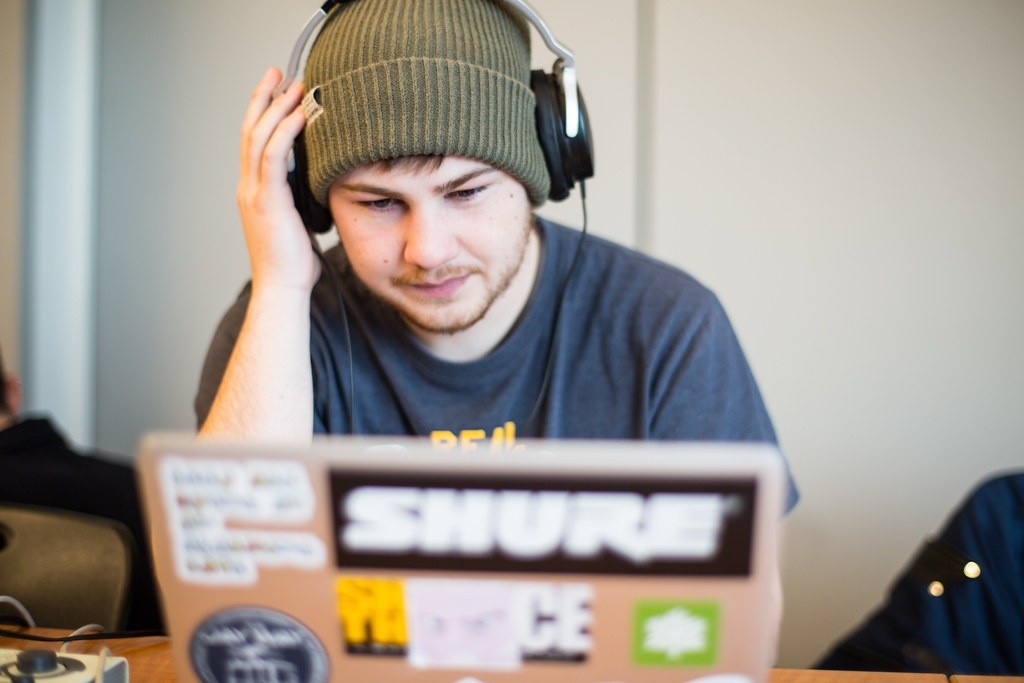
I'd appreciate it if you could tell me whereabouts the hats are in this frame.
[293,0,550,207]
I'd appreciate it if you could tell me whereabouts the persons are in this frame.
[193,0,799,520]
[811,466,1024,676]
[0,355,167,637]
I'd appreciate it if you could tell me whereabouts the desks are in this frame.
[0,625,1024,683]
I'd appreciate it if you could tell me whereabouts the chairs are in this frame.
[0,503,132,633]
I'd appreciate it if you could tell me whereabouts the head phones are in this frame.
[282,0,596,235]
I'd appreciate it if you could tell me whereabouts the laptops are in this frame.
[134,429,784,683]
